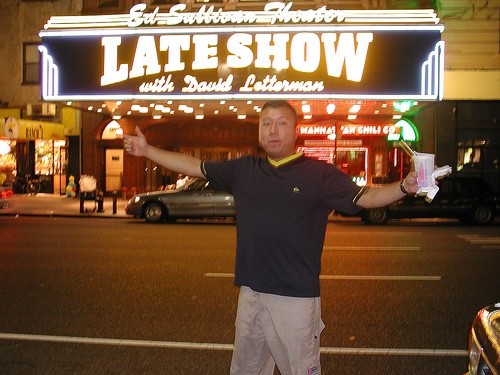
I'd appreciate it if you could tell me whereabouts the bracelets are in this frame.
[400,178,416,197]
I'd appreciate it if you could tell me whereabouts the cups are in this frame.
[412,154,435,192]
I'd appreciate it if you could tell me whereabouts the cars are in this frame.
[0,186,14,209]
[125,167,236,224]
[467,302,500,374]
[338,170,500,224]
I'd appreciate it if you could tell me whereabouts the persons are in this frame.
[116,101,449,375]
